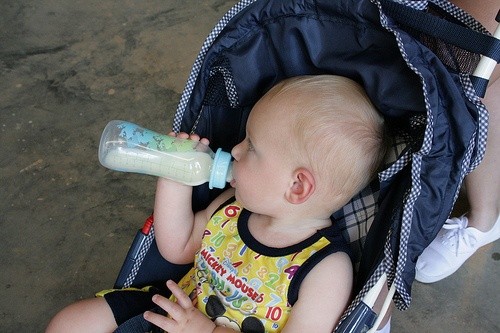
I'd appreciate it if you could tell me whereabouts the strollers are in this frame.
[113,0,500,333]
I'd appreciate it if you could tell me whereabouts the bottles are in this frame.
[98,119,233,189]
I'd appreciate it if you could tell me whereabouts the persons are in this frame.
[414,0,500,284]
[45,74,386,333]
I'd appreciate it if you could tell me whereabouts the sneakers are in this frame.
[415,209,500,283]
